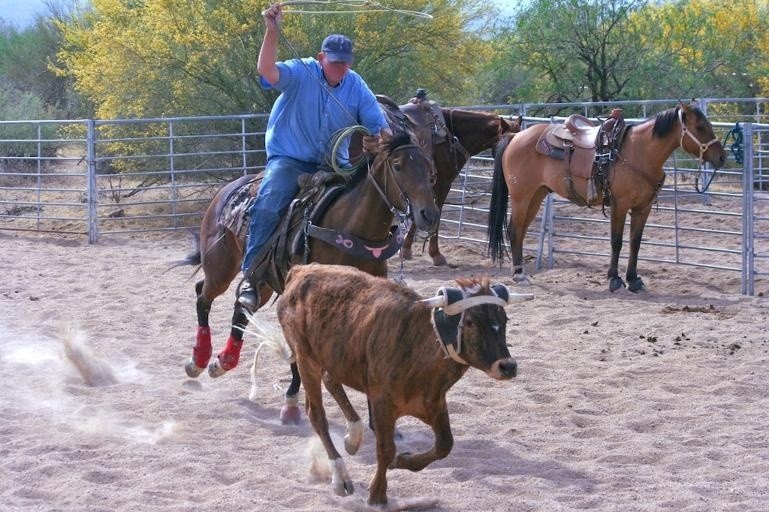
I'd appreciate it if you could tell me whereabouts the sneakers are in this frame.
[238,277,258,310]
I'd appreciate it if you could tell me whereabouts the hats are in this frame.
[322,33,354,64]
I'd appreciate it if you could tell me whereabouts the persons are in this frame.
[235,4,389,308]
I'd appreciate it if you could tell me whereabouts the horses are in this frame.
[160,128,442,443]
[485,95,727,298]
[401,107,523,266]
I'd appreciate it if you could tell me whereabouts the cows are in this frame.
[233,261,535,510]
